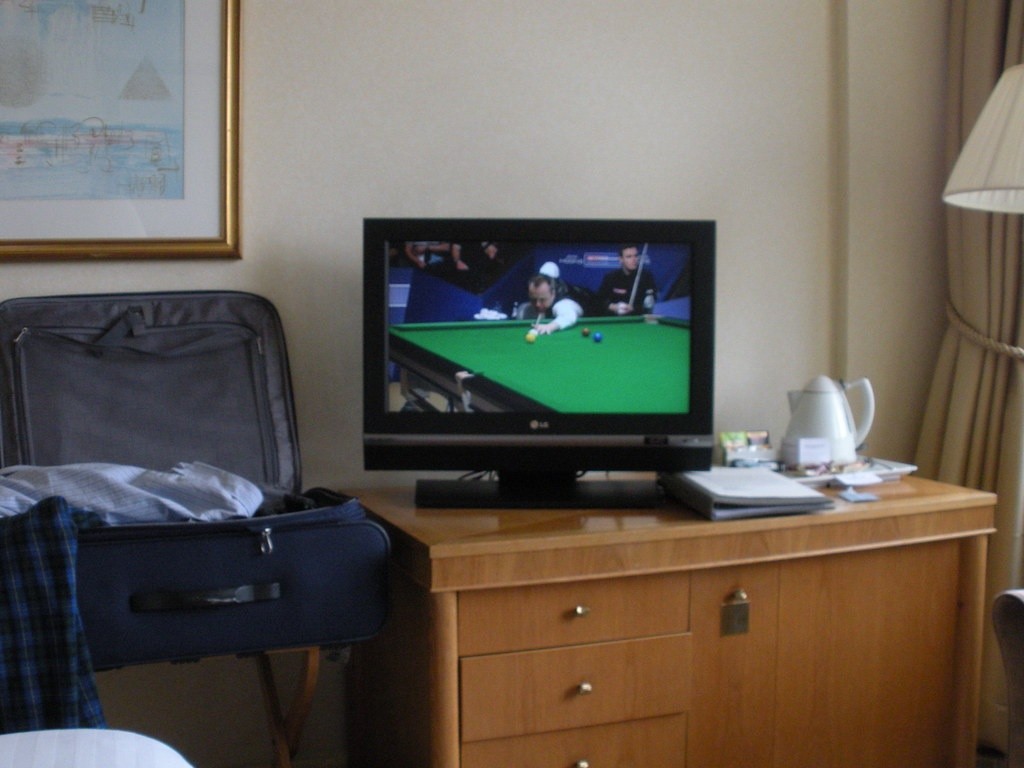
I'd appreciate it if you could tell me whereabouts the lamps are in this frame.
[941,64,1024,213]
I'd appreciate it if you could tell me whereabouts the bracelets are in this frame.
[455,260,460,263]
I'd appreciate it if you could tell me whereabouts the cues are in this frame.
[535,312,542,324]
[629,243,648,303]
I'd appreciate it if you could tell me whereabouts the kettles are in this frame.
[781,375,876,465]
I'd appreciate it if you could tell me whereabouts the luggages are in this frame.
[0,291,394,671]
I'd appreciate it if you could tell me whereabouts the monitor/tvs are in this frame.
[362,217,716,510]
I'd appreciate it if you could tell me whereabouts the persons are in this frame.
[595,242,658,317]
[528,262,605,335]
[404,241,537,294]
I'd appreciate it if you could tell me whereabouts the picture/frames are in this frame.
[0,0,241,261]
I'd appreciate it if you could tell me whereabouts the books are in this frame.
[657,463,837,521]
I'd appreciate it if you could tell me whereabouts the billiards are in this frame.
[526,329,538,342]
[582,328,590,336]
[593,332,603,342]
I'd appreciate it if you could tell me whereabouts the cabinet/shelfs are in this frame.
[348,470,998,768]
